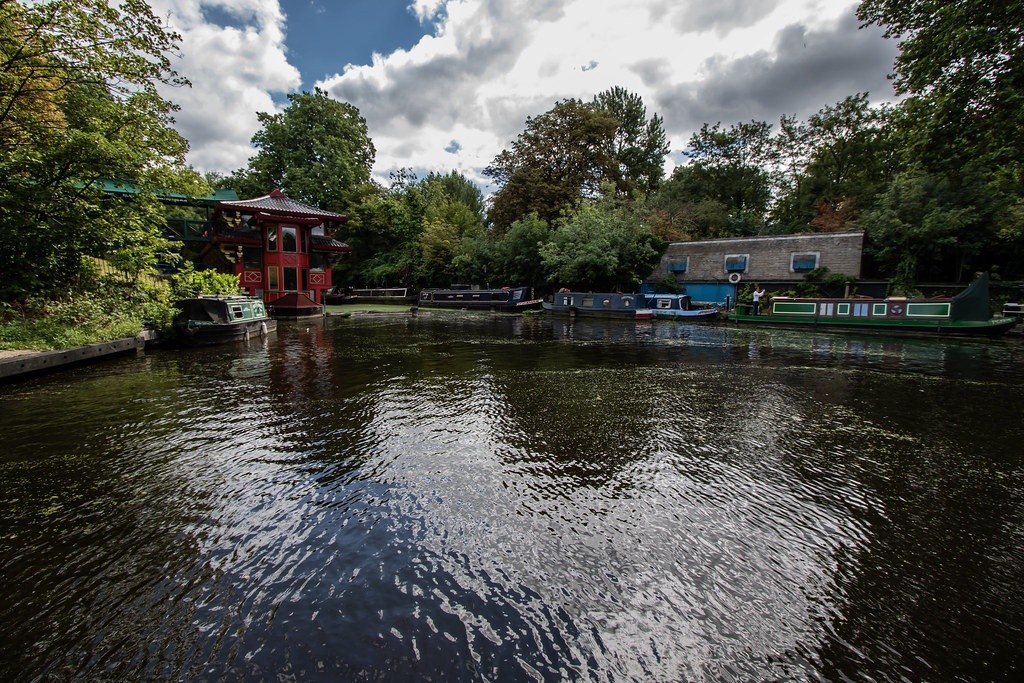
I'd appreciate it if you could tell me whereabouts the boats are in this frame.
[727,270,1019,338]
[417,286,544,311]
[541,290,720,323]
[174,291,278,346]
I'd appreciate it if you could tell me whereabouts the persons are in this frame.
[753,286,766,315]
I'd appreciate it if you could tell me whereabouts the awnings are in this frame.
[794,255,816,268]
[725,256,746,270]
[670,258,687,270]
